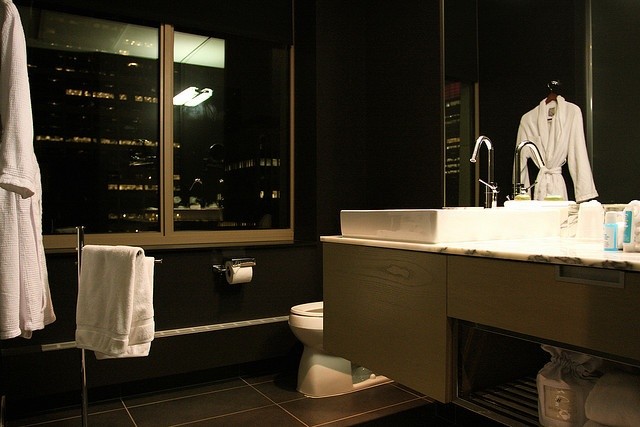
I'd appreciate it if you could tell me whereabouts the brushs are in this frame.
[233,258,256,270]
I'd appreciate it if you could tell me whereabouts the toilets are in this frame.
[288,301,395,399]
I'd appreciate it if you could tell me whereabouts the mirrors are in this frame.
[442,0,640,207]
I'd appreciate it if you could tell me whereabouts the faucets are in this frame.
[514,140,546,199]
[469,136,500,207]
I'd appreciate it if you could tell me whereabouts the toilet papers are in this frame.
[225,261,253,285]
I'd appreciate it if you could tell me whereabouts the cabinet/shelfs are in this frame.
[446,248,639,427]
[321,235,451,404]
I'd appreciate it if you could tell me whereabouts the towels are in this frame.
[94,256,155,360]
[76,244,154,358]
[582,369,639,427]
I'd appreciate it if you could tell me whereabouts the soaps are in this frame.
[513,195,532,201]
[546,195,563,201]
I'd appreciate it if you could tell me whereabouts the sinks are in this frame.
[339,208,561,244]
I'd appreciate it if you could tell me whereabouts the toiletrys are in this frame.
[602,210,619,251]
[575,198,640,252]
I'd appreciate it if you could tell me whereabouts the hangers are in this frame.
[538,79,563,109]
